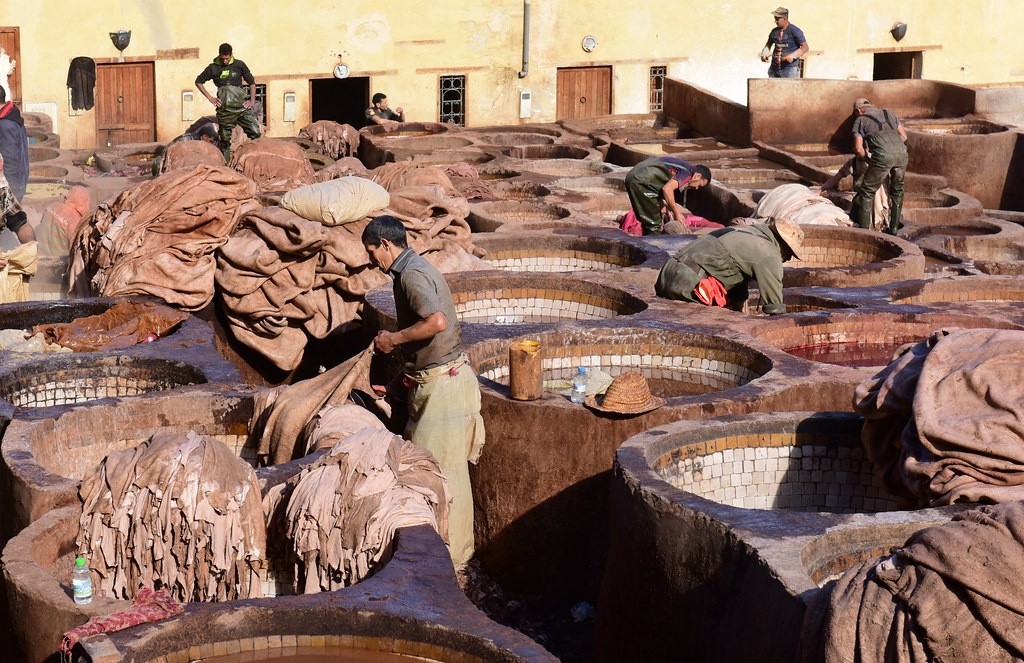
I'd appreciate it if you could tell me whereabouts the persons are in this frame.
[0,86,30,204]
[194,43,261,166]
[655,217,804,315]
[366,93,405,125]
[761,7,809,78]
[152,127,220,177]
[361,215,482,572]
[824,98,909,234]
[624,156,712,235]
[0,154,38,270]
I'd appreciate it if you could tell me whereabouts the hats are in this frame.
[771,6,789,17]
[853,97,871,114]
[771,217,805,262]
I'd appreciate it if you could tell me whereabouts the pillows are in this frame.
[279,175,390,226]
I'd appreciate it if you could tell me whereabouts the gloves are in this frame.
[760,47,769,63]
[784,49,803,62]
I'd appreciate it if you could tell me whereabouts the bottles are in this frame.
[72,558,93,604]
[571,367,588,403]
